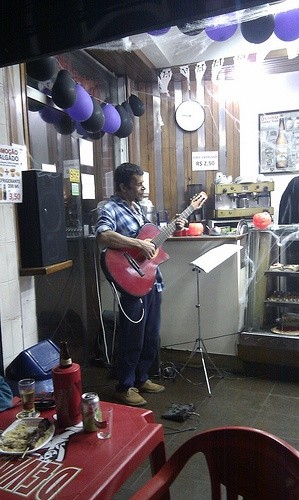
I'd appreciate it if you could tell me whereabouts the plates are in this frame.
[0,417,55,456]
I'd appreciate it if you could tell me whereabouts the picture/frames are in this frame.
[258,109,299,175]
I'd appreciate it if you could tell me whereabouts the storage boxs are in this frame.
[6,338,61,381]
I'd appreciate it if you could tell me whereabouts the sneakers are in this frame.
[115,387,147,406]
[139,380,165,393]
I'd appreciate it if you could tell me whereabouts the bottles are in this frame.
[52,341,84,427]
[276,118,288,168]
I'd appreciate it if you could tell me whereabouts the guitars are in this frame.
[99,190,208,298]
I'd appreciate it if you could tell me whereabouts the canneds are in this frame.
[81,392,102,432]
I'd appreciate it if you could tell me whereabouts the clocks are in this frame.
[174,100,206,133]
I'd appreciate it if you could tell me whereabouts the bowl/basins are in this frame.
[209,228,222,236]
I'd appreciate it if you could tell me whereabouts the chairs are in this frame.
[127,425,299,500]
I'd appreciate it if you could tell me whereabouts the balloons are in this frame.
[240,3,274,44]
[274,8,299,42]
[25,55,146,141]
[177,19,204,36]
[147,27,170,36]
[203,12,238,42]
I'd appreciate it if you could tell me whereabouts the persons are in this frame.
[94,163,189,406]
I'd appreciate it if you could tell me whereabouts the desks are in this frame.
[0,399,171,500]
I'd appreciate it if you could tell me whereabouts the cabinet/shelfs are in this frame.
[237,224,299,378]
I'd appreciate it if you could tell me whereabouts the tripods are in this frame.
[178,244,238,397]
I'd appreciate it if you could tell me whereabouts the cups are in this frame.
[92,404,113,441]
[17,379,36,417]
[189,222,204,236]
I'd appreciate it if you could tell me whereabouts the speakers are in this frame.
[15,170,69,269]
[6,337,61,383]
